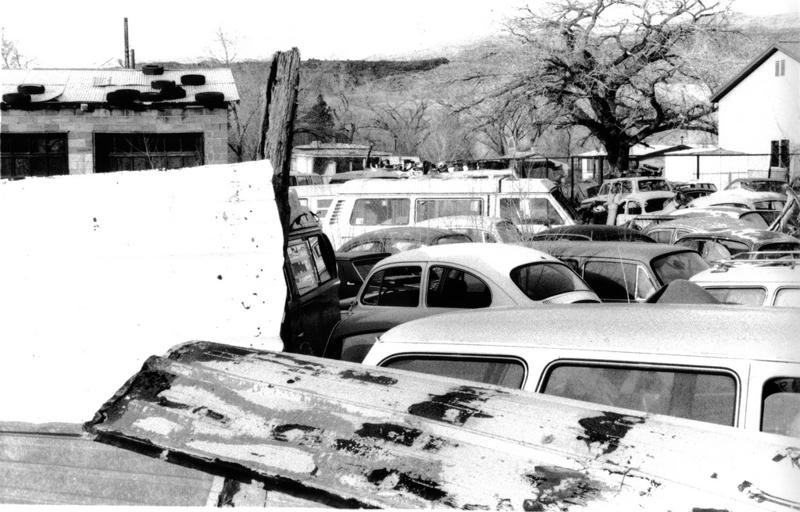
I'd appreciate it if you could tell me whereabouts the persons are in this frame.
[367,154,489,175]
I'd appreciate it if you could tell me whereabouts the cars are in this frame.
[348,241,599,304]
[278,151,800,363]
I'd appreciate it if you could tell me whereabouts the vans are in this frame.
[318,174,585,256]
[359,301,799,442]
[523,239,710,302]
